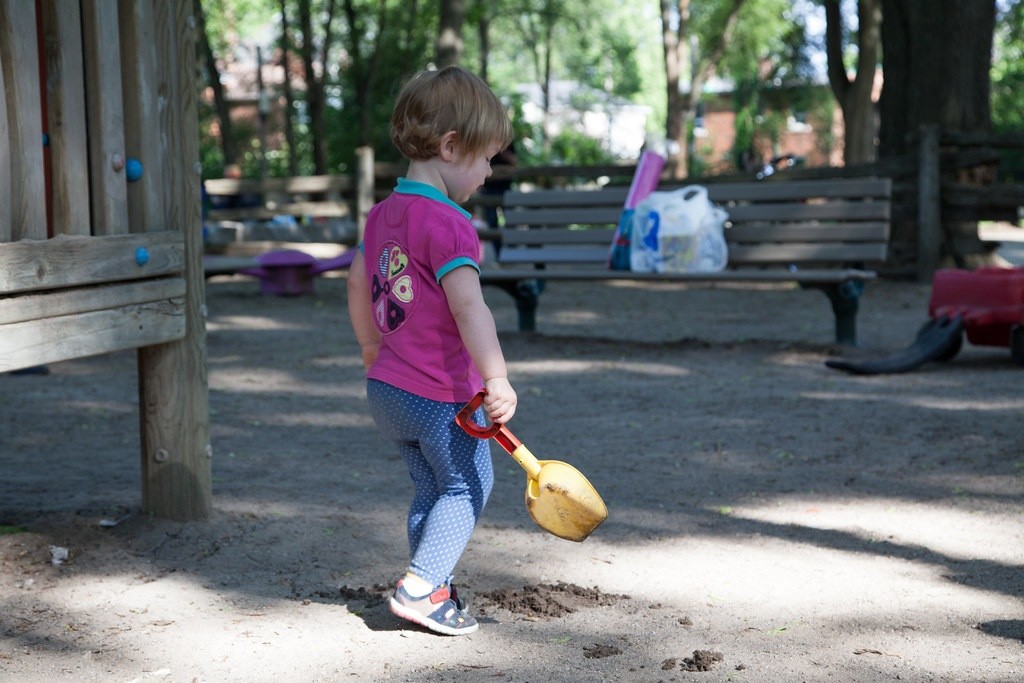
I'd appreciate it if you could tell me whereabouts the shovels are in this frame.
[454,387,609,543]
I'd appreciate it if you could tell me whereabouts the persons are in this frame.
[347,67,518,635]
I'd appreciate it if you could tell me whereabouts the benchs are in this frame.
[479,176,895,347]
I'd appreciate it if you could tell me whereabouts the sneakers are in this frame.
[386,577,479,635]
[396,566,469,614]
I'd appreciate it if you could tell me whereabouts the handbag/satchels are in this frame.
[628,183,729,277]
